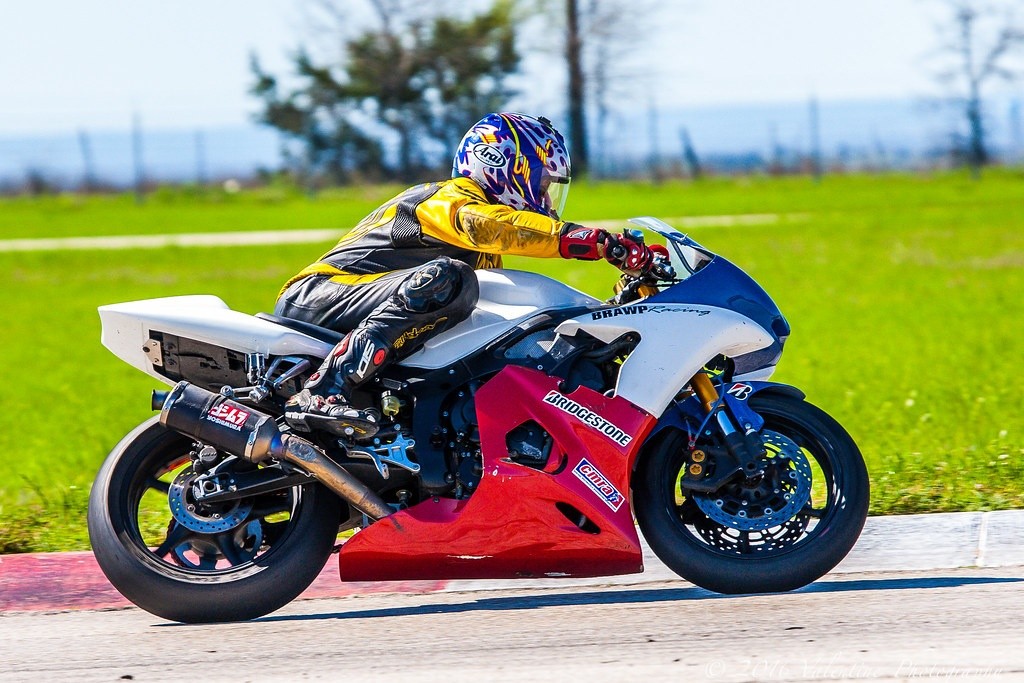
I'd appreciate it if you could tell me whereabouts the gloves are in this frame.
[558,220,655,276]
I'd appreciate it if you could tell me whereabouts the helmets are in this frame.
[451,111,572,221]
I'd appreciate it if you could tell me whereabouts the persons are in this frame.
[274,111,654,440]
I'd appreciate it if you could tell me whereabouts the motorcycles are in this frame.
[83,215,872,625]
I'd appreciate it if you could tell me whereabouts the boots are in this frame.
[284,328,399,440]
